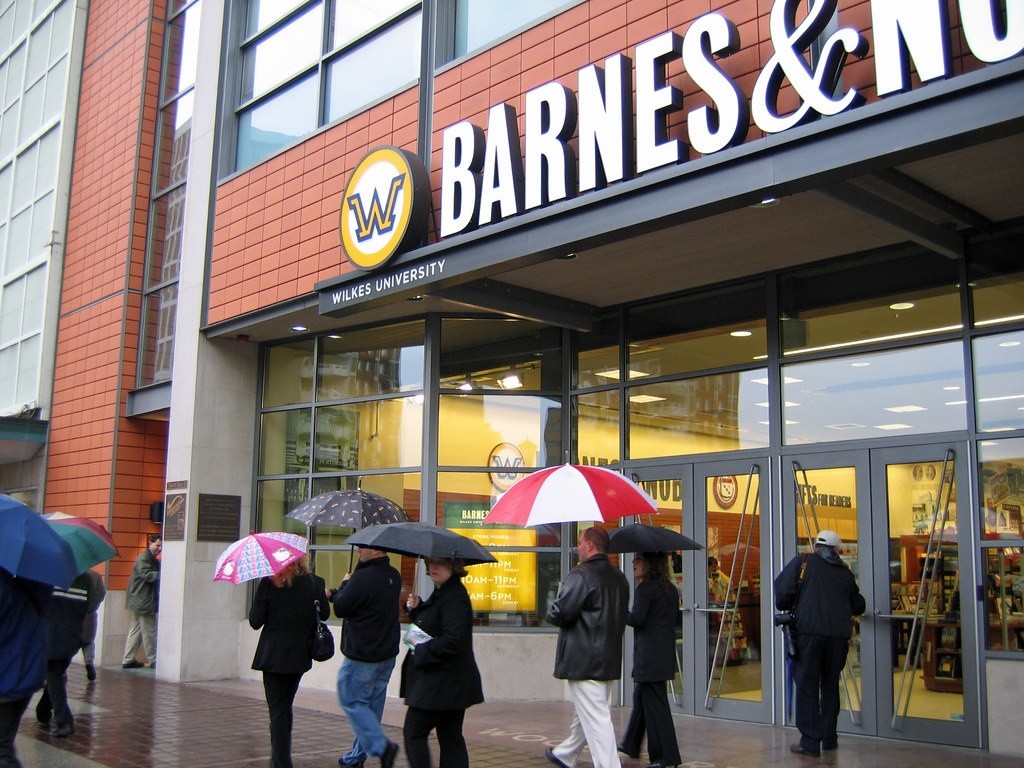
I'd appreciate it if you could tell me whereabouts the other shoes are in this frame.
[38,721,50,728]
[617,743,638,760]
[647,762,678,768]
[51,723,74,736]
[381,741,399,768]
[546,748,569,768]
[123,660,144,668]
[338,758,363,768]
[86,663,96,681]
[151,662,156,668]
[821,743,837,750]
[791,744,817,757]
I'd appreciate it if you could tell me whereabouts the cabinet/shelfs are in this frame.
[918,618,1024,693]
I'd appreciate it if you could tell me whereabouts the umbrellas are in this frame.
[574,523,704,554]
[481,462,660,571]
[281,476,413,574]
[213,529,310,586]
[343,521,499,593]
[0,493,80,591]
[42,511,121,575]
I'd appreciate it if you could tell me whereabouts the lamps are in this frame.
[497,365,525,389]
[149,500,162,524]
[454,372,479,398]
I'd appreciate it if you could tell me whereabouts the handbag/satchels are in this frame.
[312,622,334,662]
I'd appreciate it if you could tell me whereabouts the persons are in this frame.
[36,572,95,736]
[122,535,163,667]
[399,555,485,768]
[772,530,865,757]
[545,527,630,768]
[248,553,331,768]
[81,569,107,681]
[0,565,49,768]
[617,554,682,768]
[324,547,402,768]
[707,556,733,594]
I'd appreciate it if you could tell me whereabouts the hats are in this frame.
[816,530,841,546]
